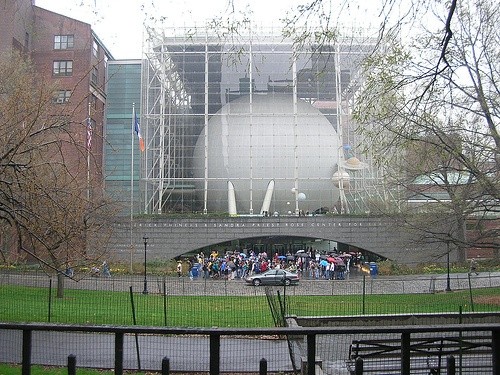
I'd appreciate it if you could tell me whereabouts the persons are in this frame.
[68,263,73,277]
[469,258,479,276]
[177,262,183,278]
[187,247,364,280]
[101,261,111,277]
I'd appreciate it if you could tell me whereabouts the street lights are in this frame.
[142,233,149,294]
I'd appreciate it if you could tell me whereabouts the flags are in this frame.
[87,117,92,148]
[135,113,145,151]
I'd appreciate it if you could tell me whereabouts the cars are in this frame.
[245,268,300,286]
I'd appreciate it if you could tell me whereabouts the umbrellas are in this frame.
[349,252,356,254]
[327,257,336,262]
[287,256,295,260]
[320,255,328,260]
[338,255,346,257]
[240,253,247,257]
[320,261,328,266]
[278,256,286,260]
[327,250,336,252]
[344,254,352,256]
[330,253,338,256]
[301,253,308,257]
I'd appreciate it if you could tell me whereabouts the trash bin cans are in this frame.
[192,265,198,276]
[370,264,376,275]
